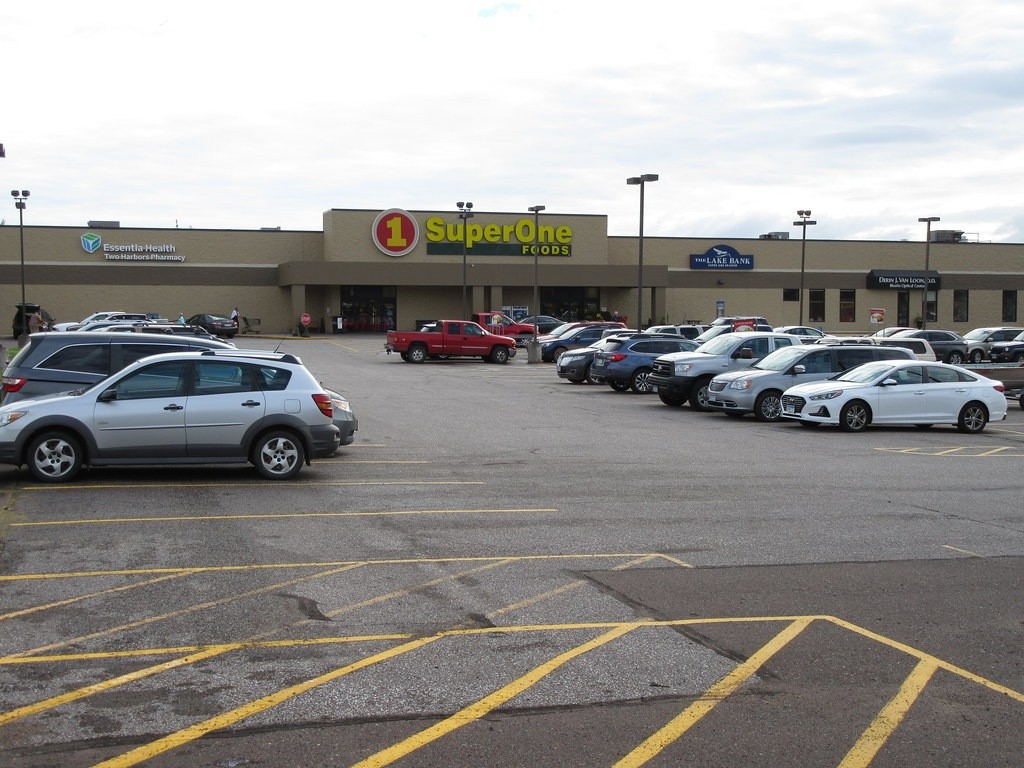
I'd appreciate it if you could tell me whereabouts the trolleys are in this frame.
[241,317,260,335]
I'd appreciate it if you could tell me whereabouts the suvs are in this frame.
[530,315,1024,423]
[0,311,358,447]
[0,348,341,484]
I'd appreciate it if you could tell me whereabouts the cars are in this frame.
[780,360,1007,434]
[185,313,238,338]
[515,316,567,334]
[12,303,56,339]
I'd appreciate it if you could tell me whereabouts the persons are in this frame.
[178,313,186,324]
[231,306,241,327]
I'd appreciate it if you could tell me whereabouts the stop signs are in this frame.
[301,313,310,325]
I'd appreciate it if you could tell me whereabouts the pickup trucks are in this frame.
[384,312,539,365]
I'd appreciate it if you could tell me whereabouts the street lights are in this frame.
[626,174,658,332]
[528,205,546,364]
[792,210,816,327]
[456,201,474,320]
[11,190,30,348]
[918,217,940,330]
